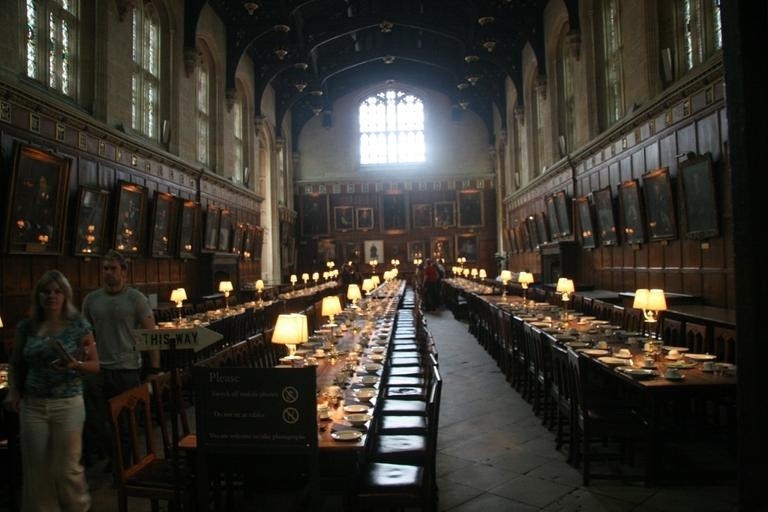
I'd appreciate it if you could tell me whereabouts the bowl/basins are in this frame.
[331,317,392,427]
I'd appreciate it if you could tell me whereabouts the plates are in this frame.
[507,301,733,379]
[332,430,362,442]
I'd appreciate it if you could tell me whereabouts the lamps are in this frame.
[311,257,534,299]
[244,0,497,117]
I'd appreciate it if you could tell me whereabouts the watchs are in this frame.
[78,360,82,367]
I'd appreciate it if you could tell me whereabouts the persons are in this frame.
[5,270,100,512]
[80,250,162,488]
[416,257,445,312]
[341,263,359,309]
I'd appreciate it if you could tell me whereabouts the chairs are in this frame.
[94,274,442,511]
[443,274,736,485]
